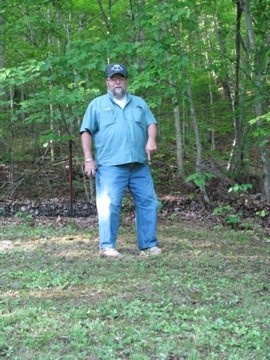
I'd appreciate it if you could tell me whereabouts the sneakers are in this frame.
[99,247,121,258]
[138,247,163,258]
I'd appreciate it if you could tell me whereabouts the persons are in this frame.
[79,64,162,259]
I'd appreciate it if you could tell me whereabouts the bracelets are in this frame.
[85,158,93,161]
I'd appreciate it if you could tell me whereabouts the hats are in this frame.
[106,64,128,79]
